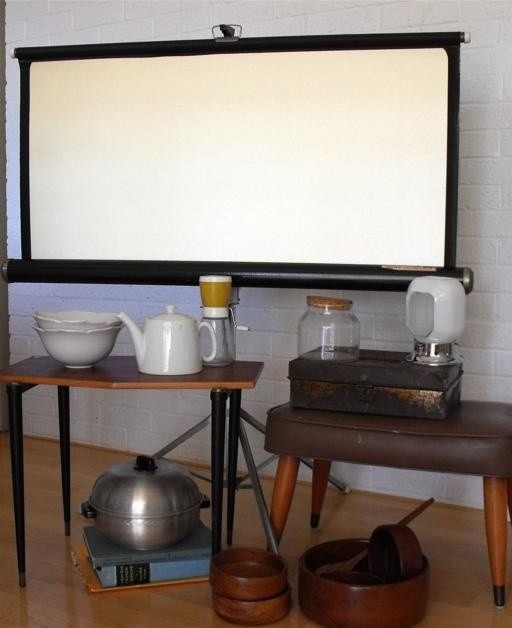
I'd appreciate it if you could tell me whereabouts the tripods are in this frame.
[149,286,350,557]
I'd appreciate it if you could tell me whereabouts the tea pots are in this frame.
[119,304,218,376]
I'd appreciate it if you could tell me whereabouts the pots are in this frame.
[80,454,212,550]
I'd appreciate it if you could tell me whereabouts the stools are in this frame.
[266,399,511,608]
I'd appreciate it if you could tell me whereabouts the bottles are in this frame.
[405,276,467,366]
[297,295,361,362]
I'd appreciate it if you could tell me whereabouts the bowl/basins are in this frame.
[31,307,128,371]
[209,523,432,628]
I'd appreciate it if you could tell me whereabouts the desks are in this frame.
[0,357,263,586]
